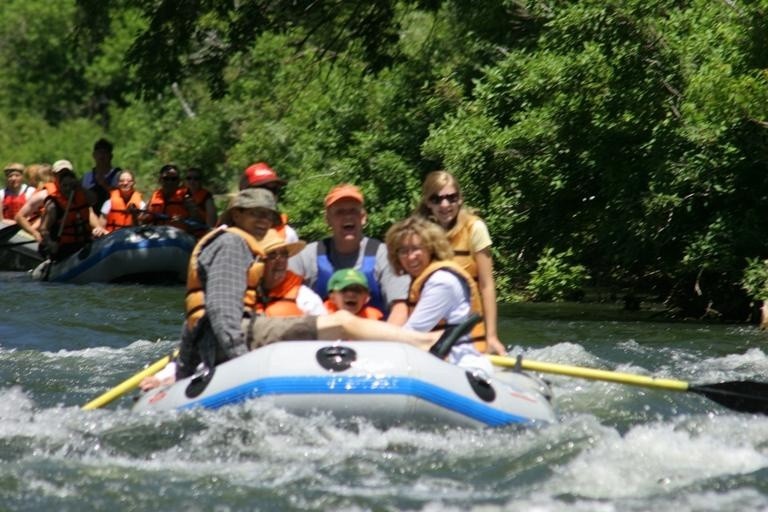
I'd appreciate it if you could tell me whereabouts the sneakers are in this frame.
[429,311,482,359]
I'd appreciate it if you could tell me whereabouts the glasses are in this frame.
[428,193,459,205]
[267,250,289,259]
[266,184,281,195]
[161,176,177,182]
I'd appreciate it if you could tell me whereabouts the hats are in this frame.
[4,163,24,174]
[239,162,277,191]
[326,268,369,293]
[322,183,364,209]
[259,228,306,257]
[222,187,282,227]
[51,160,74,173]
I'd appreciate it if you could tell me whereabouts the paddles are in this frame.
[486,350,768,417]
[32,189,76,281]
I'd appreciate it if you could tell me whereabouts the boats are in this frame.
[0,219,199,287]
[130,339,556,433]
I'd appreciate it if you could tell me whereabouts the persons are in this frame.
[185,188,480,389]
[385,215,494,383]
[323,268,383,320]
[286,184,411,328]
[162,187,480,383]
[259,228,328,316]
[239,164,299,244]
[1,138,217,253]
[412,171,506,357]
